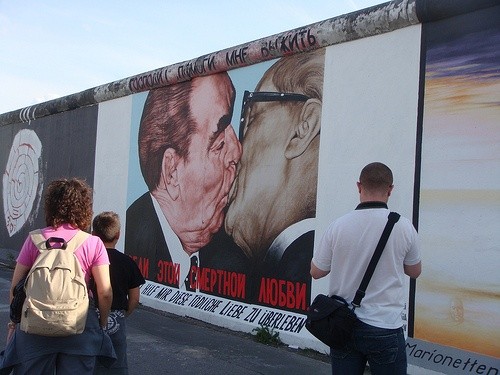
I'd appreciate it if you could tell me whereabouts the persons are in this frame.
[310,162,421,375]
[90,210,146,375]
[0,176,118,375]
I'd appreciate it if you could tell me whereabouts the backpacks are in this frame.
[19,228,90,337]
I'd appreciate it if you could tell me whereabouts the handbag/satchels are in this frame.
[305,294,357,350]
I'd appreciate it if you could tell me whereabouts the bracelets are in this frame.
[8,323,16,329]
